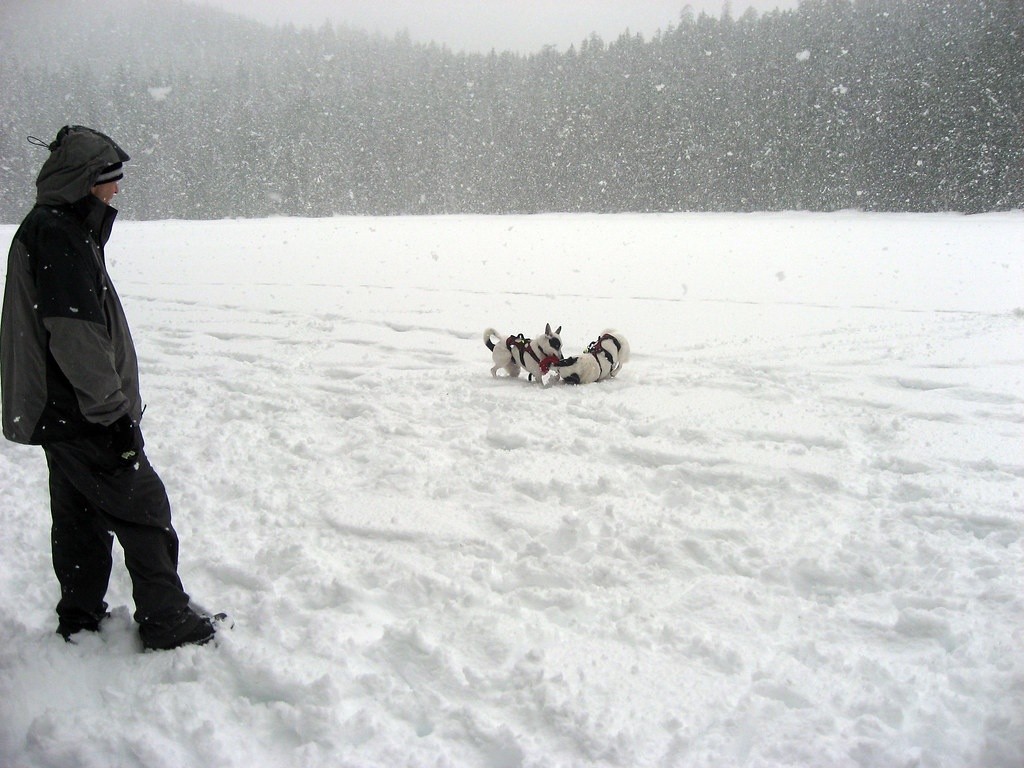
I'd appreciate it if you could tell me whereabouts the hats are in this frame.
[94,161,122,186]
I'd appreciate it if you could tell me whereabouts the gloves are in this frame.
[107,413,140,469]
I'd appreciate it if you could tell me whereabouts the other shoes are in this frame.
[142,609,234,652]
[56,612,111,642]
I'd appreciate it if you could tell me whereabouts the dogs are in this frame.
[482,323,631,388]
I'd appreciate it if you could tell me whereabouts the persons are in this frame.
[0,125,234,651]
